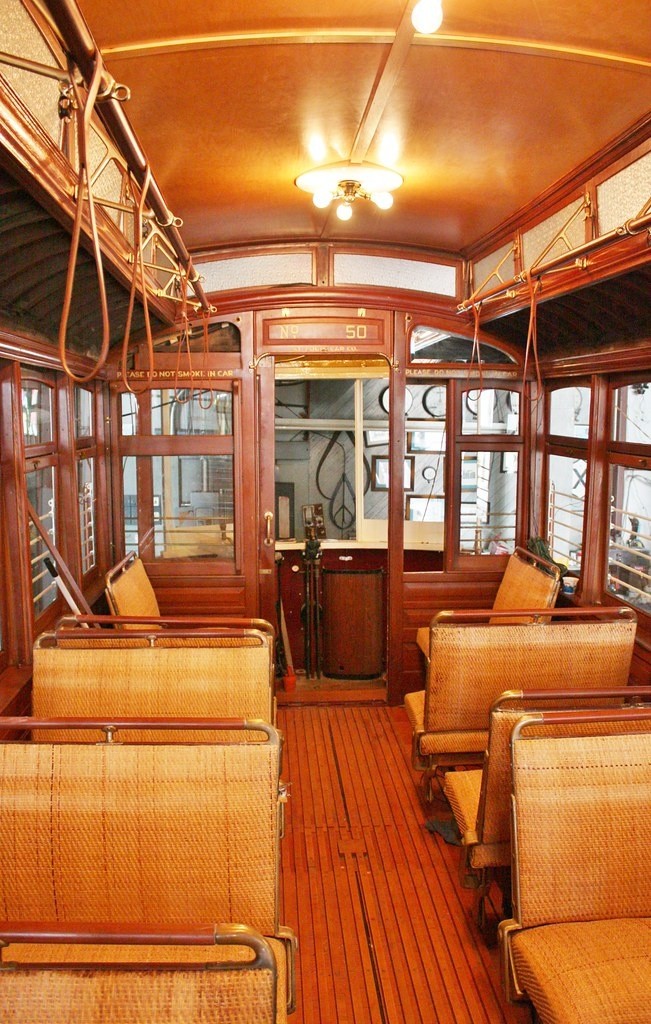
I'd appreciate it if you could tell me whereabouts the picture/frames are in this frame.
[363,430,389,448]
[371,455,415,492]
[407,417,446,455]
[406,494,446,522]
[443,456,478,493]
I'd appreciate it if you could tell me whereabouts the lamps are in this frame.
[294,159,405,221]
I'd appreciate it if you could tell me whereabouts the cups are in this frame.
[562,577,579,595]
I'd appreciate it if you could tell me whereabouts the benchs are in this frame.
[417,545,560,660]
[32,629,270,741]
[495,708,651,1024]
[0,920,278,1024]
[0,716,297,1015]
[55,614,276,639]
[439,685,651,937]
[402,607,640,804]
[104,549,162,630]
